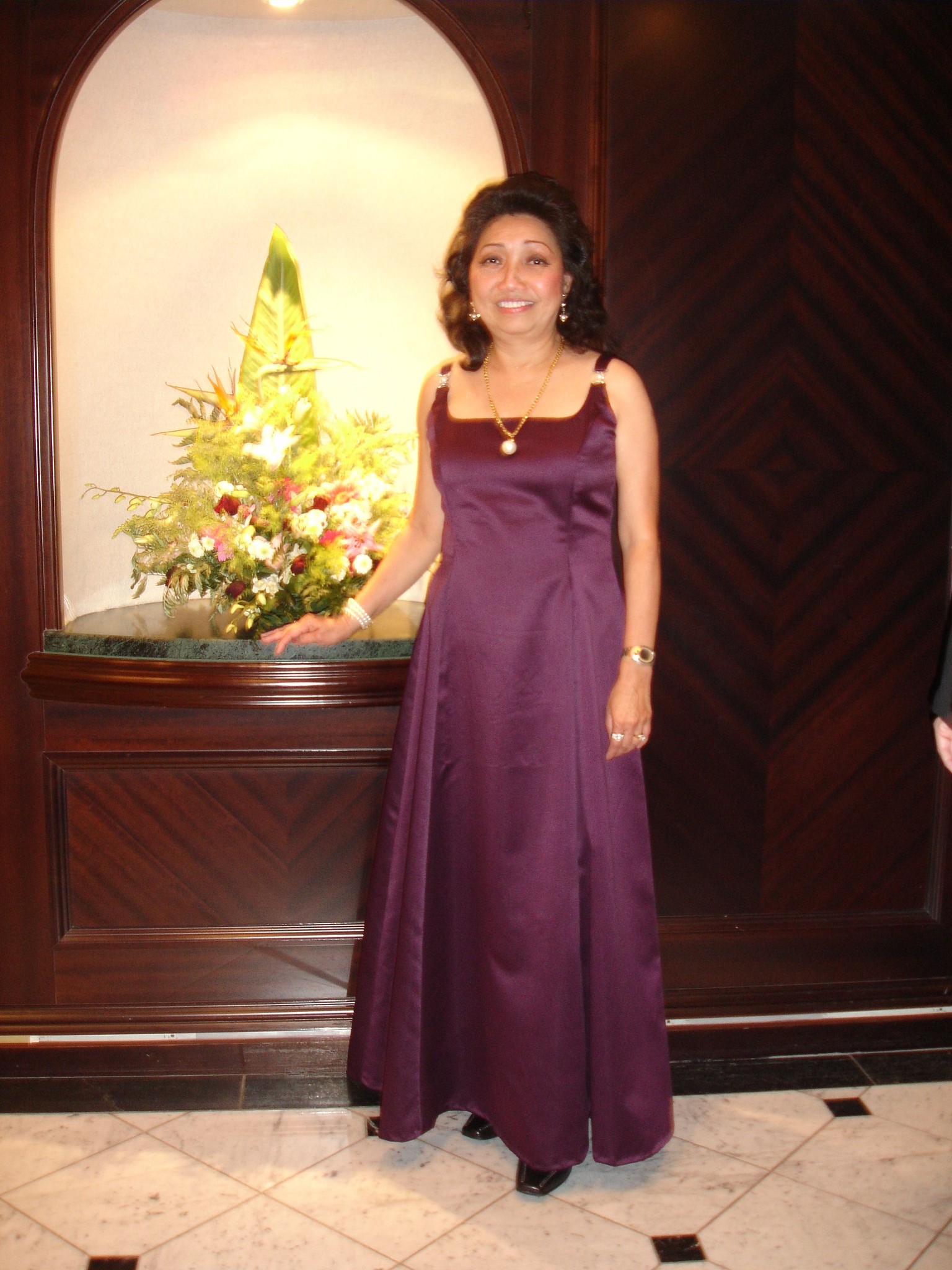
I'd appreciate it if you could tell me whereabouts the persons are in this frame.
[932,633,952,768]
[261,172,677,1197]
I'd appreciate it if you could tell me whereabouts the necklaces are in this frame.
[482,335,568,455]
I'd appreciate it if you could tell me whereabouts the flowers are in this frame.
[78,359,420,642]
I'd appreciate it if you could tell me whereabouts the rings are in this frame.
[611,733,624,741]
[633,734,646,741]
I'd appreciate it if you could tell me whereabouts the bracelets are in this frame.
[343,595,374,632]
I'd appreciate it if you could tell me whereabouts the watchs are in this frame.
[621,644,657,665]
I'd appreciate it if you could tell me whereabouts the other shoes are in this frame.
[461,1112,498,1140]
[515,1158,572,1196]
